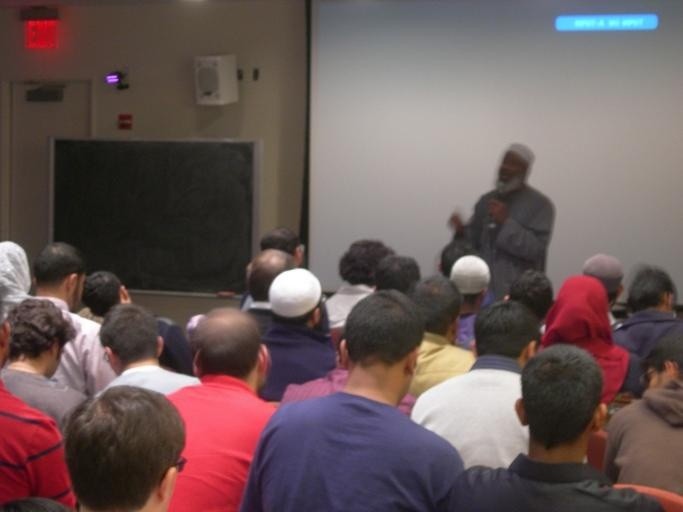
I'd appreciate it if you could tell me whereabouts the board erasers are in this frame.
[217,291,235,297]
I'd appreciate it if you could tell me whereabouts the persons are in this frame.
[0,241,275,511]
[449,143,554,306]
[237,226,466,512]
[409,239,682,512]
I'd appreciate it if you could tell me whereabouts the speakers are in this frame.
[195,55,239,106]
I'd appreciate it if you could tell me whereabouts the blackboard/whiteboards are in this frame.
[48,137,263,301]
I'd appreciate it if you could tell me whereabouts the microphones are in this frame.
[487,190,500,232]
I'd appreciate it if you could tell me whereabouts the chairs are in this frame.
[587,429,606,470]
[615,484,682,512]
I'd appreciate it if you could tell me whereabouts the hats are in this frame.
[268,269,322,319]
[583,255,624,295]
[451,255,491,293]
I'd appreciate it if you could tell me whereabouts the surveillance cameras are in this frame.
[105,70,129,89]
